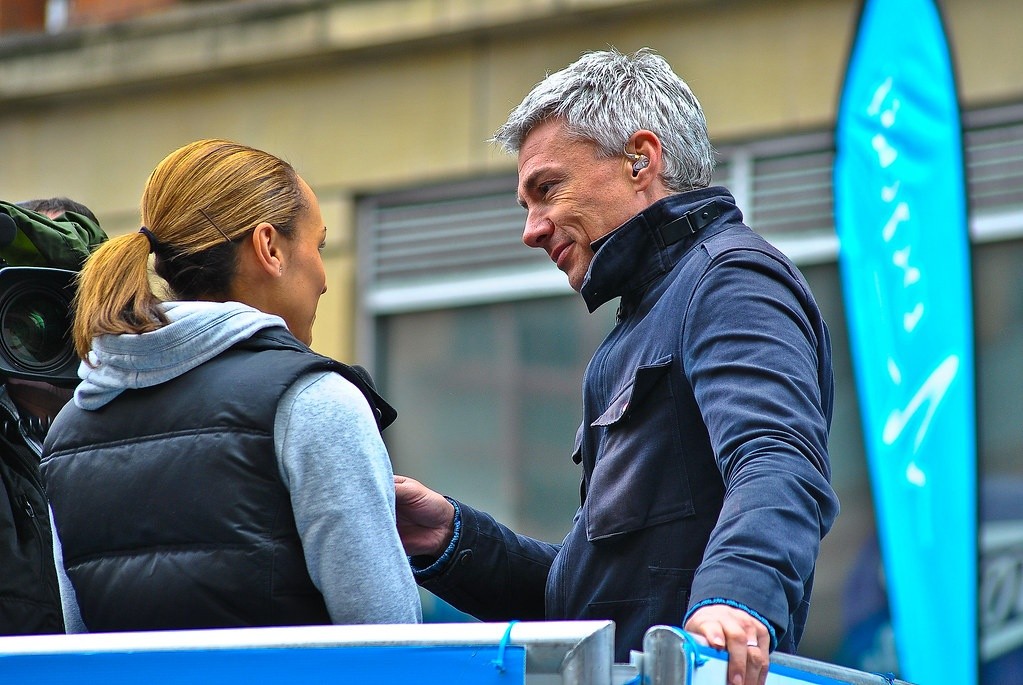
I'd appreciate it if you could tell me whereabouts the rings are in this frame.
[747,641,757,647]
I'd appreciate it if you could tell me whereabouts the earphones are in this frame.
[623,140,650,172]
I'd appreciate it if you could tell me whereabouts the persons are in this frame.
[38,138,424,634]
[394,51,841,685]
[17,198,101,229]
